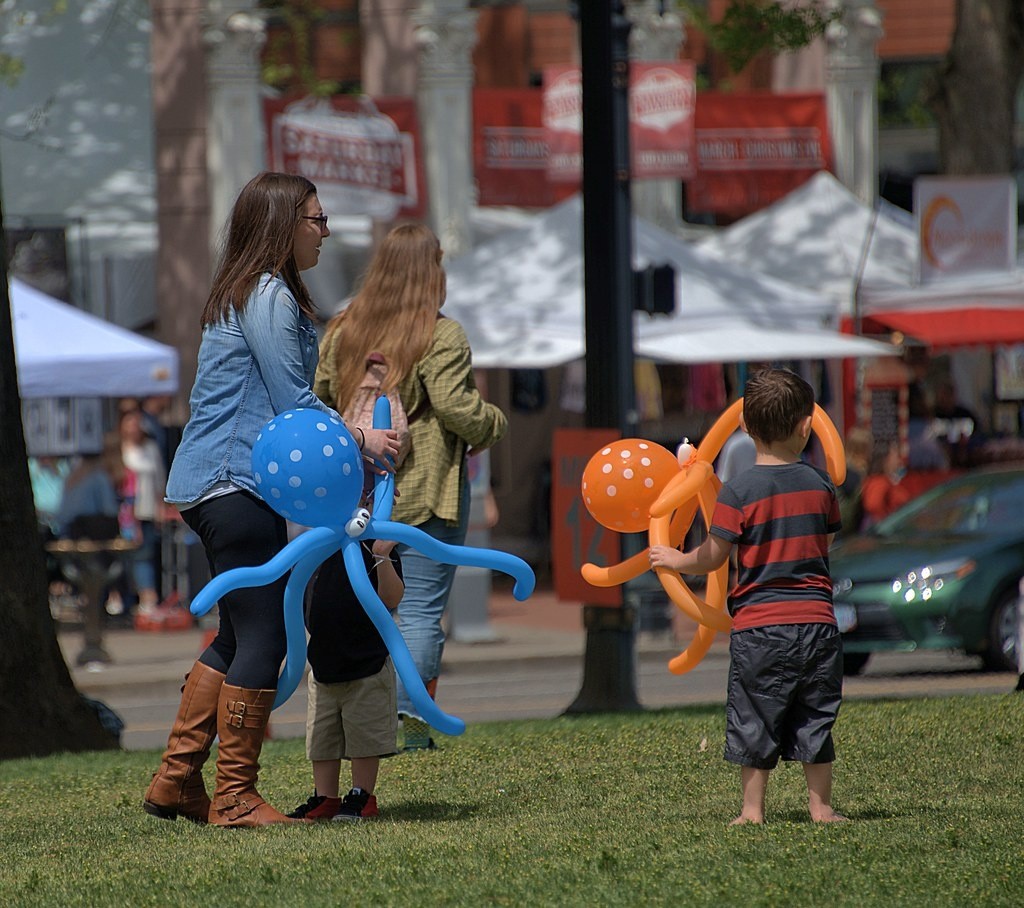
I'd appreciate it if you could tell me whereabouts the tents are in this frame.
[7,277,179,401]
[330,170,1024,372]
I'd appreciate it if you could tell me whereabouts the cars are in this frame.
[828,462,1024,674]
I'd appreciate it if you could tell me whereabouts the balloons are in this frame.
[580,381,846,672]
[189,396,536,734]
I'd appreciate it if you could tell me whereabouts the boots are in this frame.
[207,682,312,828]
[142,657,226,826]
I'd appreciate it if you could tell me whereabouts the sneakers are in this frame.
[333,787,380,823]
[287,791,341,822]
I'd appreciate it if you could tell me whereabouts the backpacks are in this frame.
[339,352,410,473]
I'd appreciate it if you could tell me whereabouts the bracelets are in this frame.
[358,426,367,452]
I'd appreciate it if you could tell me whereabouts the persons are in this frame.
[288,460,405,819]
[930,383,985,471]
[144,170,403,827]
[648,368,853,828]
[314,220,510,749]
[27,397,175,617]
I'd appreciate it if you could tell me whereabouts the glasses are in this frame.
[293,215,328,231]
